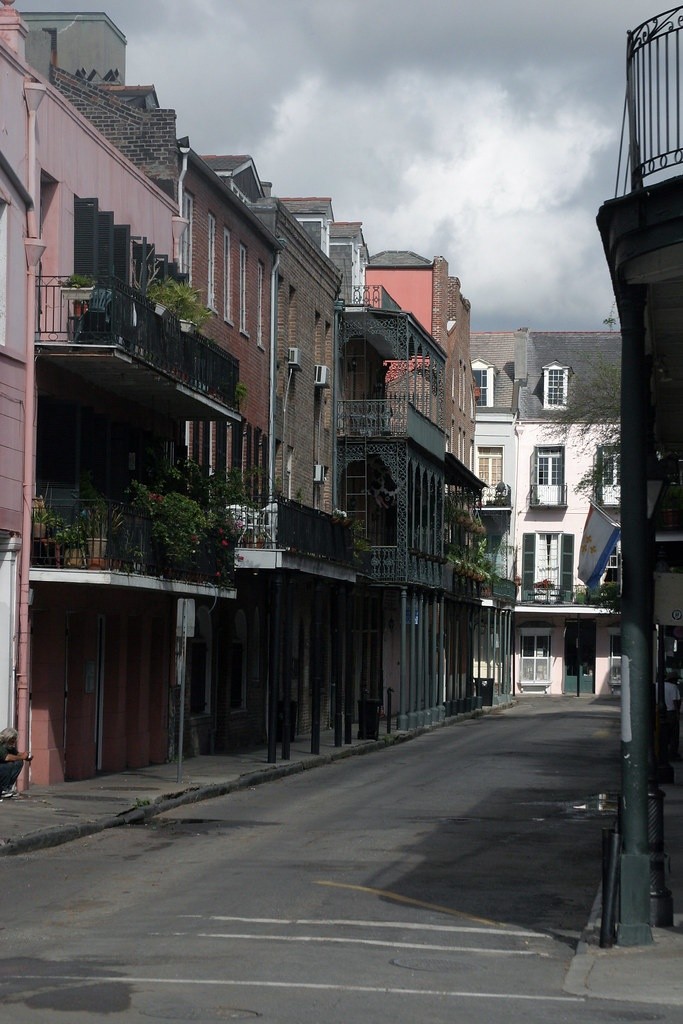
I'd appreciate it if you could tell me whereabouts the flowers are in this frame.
[332,508,347,520]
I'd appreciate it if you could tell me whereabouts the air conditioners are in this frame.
[289,348,301,370]
[313,464,324,484]
[314,365,330,384]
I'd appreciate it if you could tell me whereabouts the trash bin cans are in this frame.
[473,678,494,706]
[357,699,383,741]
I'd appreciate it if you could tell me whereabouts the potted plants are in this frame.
[453,508,484,534]
[445,555,491,582]
[51,523,84,568]
[150,277,214,336]
[244,529,267,549]
[72,300,89,315]
[122,543,145,573]
[58,275,96,299]
[31,481,61,538]
[71,492,127,559]
[342,515,355,527]
[533,579,555,589]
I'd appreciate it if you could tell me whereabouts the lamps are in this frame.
[447,321,457,336]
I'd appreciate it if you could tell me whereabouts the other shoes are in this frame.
[9,790,19,796]
[0,791,12,798]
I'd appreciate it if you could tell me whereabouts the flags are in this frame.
[577,502,621,591]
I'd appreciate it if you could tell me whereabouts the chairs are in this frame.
[226,504,257,547]
[72,288,116,343]
[236,499,288,549]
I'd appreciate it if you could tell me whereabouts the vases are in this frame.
[111,560,122,570]
[332,517,342,523]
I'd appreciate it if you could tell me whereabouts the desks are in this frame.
[68,316,83,339]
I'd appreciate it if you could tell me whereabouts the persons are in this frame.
[0,729,33,797]
[655,682,681,760]
